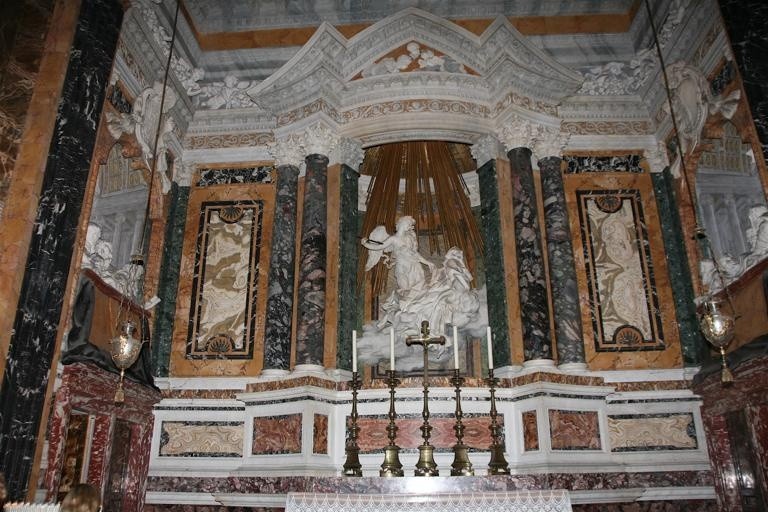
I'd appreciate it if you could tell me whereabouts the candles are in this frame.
[351,325,395,372]
[450,325,495,369]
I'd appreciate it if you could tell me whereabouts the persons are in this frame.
[183,65,206,98]
[59,483,102,512]
[202,72,240,108]
[359,213,438,329]
[377,246,483,357]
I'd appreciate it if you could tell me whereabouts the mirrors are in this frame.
[78,138,169,318]
[668,80,768,315]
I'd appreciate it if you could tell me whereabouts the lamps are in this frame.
[106,1,148,405]
[645,0,742,387]
